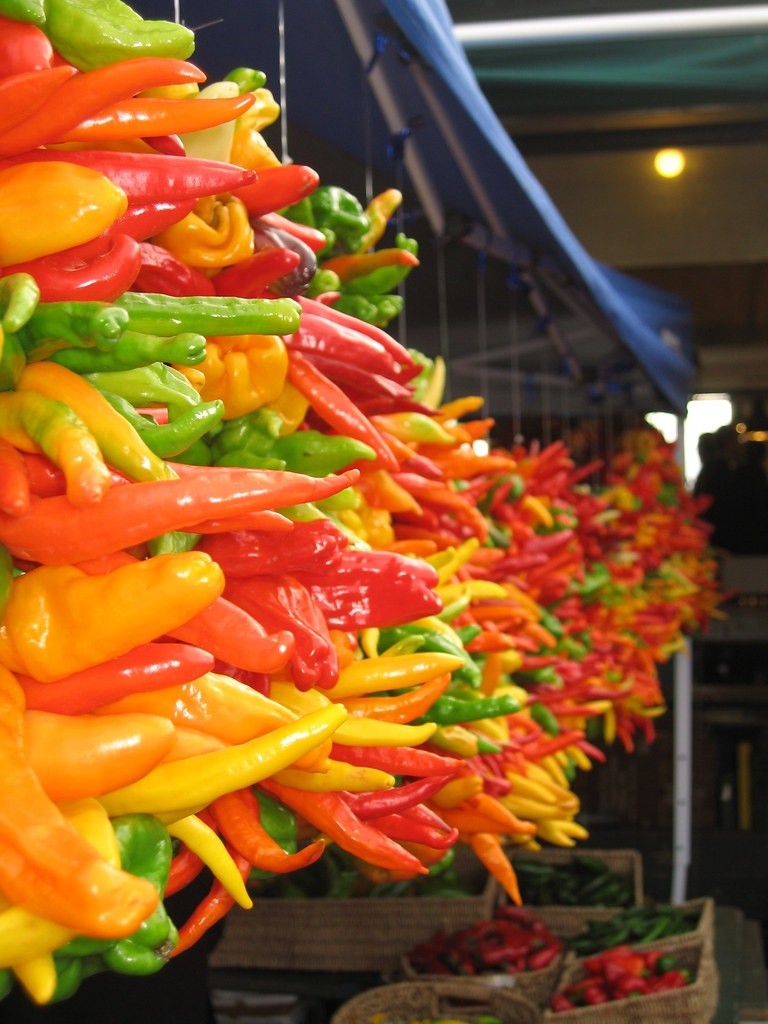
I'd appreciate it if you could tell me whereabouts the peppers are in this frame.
[0,0,736,1024]
